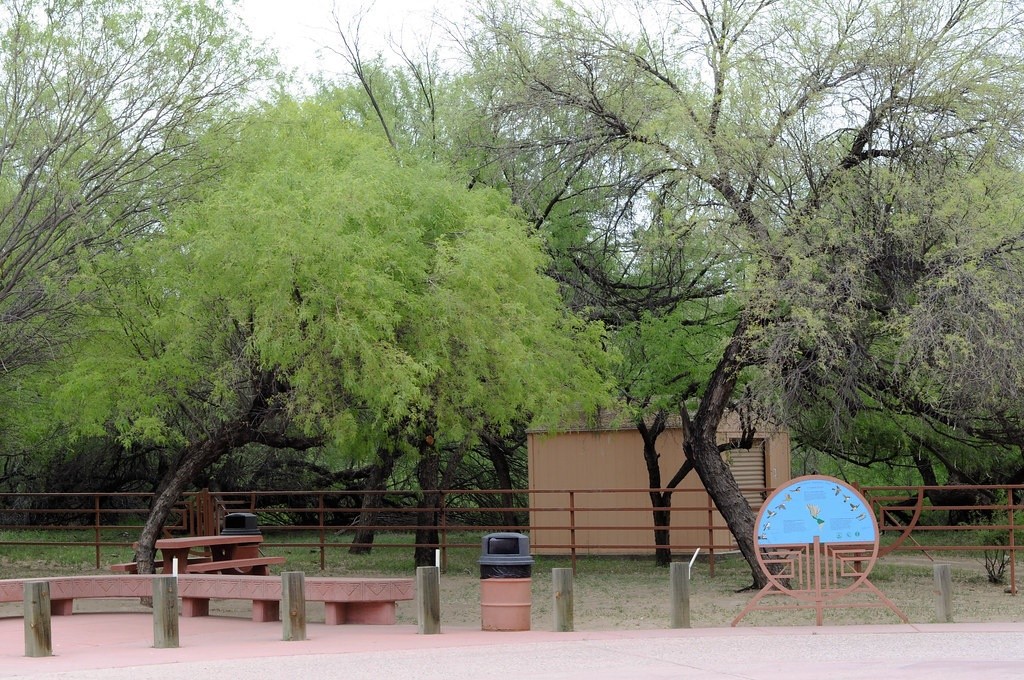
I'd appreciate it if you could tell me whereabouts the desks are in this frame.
[133,535,265,574]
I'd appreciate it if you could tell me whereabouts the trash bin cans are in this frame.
[479,532,535,632]
[220,513,260,572]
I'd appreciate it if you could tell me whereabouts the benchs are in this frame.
[112,558,212,574]
[180,556,285,619]
